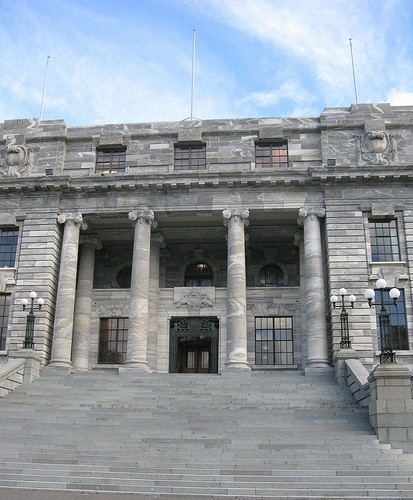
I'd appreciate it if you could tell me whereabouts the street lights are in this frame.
[330,287,356,349]
[365,279,401,364]
[21,292,44,350]
